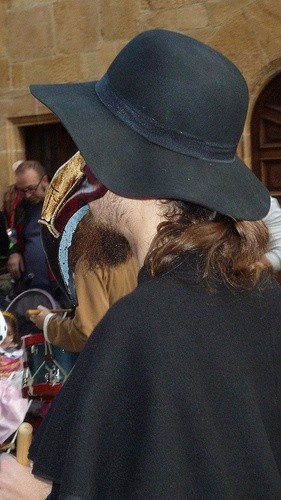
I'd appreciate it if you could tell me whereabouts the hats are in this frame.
[29,29,271,222]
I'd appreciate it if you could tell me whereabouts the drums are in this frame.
[19,331,79,399]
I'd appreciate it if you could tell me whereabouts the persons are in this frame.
[7,160,73,382]
[0,310,35,446]
[25,28,280,500]
[26,247,138,354]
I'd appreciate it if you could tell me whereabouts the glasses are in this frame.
[14,173,46,195]
